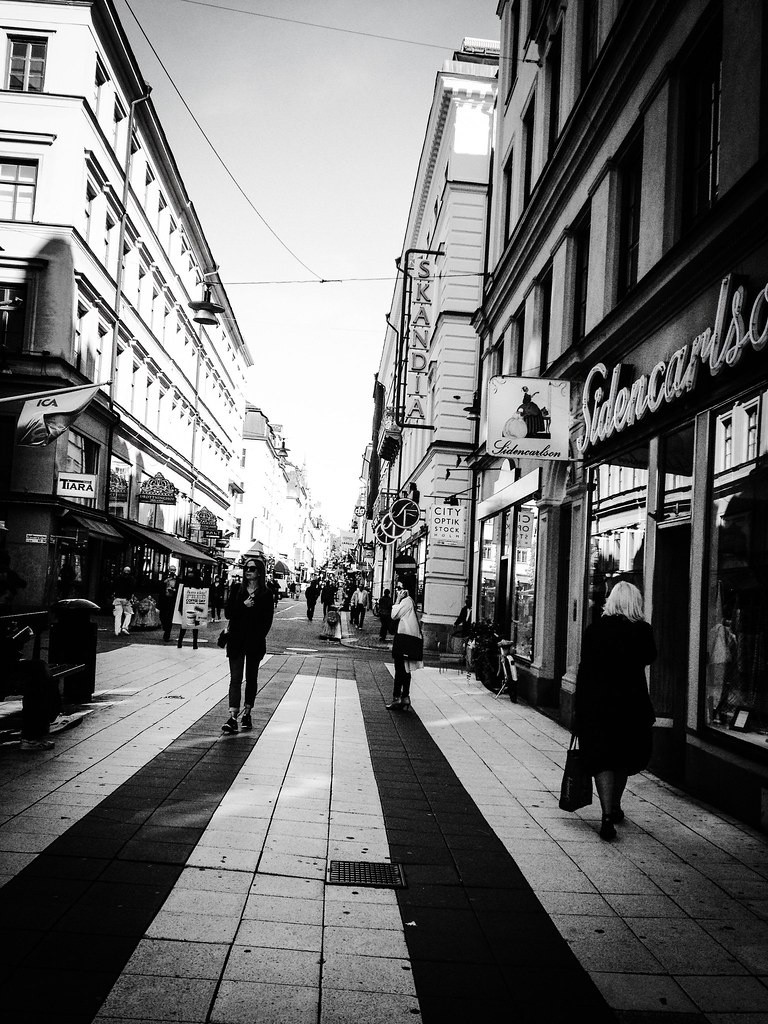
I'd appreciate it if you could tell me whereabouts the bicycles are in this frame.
[373,598,379,617]
[476,630,518,704]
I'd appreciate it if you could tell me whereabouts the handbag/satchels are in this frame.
[558,729,593,812]
[391,634,424,662]
[217,628,229,648]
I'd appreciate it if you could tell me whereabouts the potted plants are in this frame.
[470,618,498,681]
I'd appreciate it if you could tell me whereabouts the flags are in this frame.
[14,384,101,449]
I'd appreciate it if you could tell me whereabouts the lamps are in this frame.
[466,413,477,420]
[445,468,501,481]
[455,454,491,468]
[443,484,480,506]
[188,285,226,325]
[275,438,291,457]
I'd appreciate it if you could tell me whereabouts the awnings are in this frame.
[122,518,217,565]
[75,515,122,541]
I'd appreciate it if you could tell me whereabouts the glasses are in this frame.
[244,566,258,573]
[395,586,405,591]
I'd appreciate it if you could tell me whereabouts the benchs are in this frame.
[0,610,86,700]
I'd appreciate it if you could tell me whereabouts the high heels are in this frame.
[385,696,410,711]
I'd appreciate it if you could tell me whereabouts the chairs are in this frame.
[438,633,469,676]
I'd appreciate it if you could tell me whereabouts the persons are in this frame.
[230,575,241,596]
[454,594,472,630]
[111,566,135,636]
[305,571,371,630]
[265,574,281,608]
[209,575,222,620]
[221,559,274,732]
[379,588,393,640]
[0,579,64,751]
[0,548,26,596]
[155,565,204,649]
[286,581,301,600]
[385,575,423,710]
[572,579,657,842]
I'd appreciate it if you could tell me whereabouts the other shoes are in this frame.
[162,636,171,642]
[222,718,238,732]
[613,808,626,825]
[240,715,252,731]
[600,821,618,844]
[121,628,130,635]
